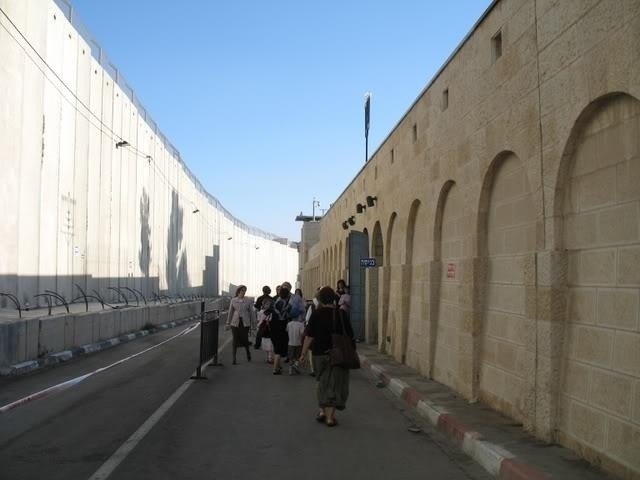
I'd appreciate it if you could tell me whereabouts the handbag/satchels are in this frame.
[261,319,273,339]
[331,334,359,369]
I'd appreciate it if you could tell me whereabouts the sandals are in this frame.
[272,365,282,375]
[316,411,326,422]
[326,416,337,427]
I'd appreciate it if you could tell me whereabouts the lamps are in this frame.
[342,221,348,230]
[348,216,355,225]
[356,204,366,213]
[366,196,377,207]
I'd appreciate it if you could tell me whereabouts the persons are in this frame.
[299,287,357,427]
[226,284,256,364]
[254,279,352,375]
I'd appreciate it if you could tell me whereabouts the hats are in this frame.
[235,284,247,294]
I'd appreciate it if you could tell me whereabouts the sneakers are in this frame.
[264,358,275,362]
[292,361,303,374]
